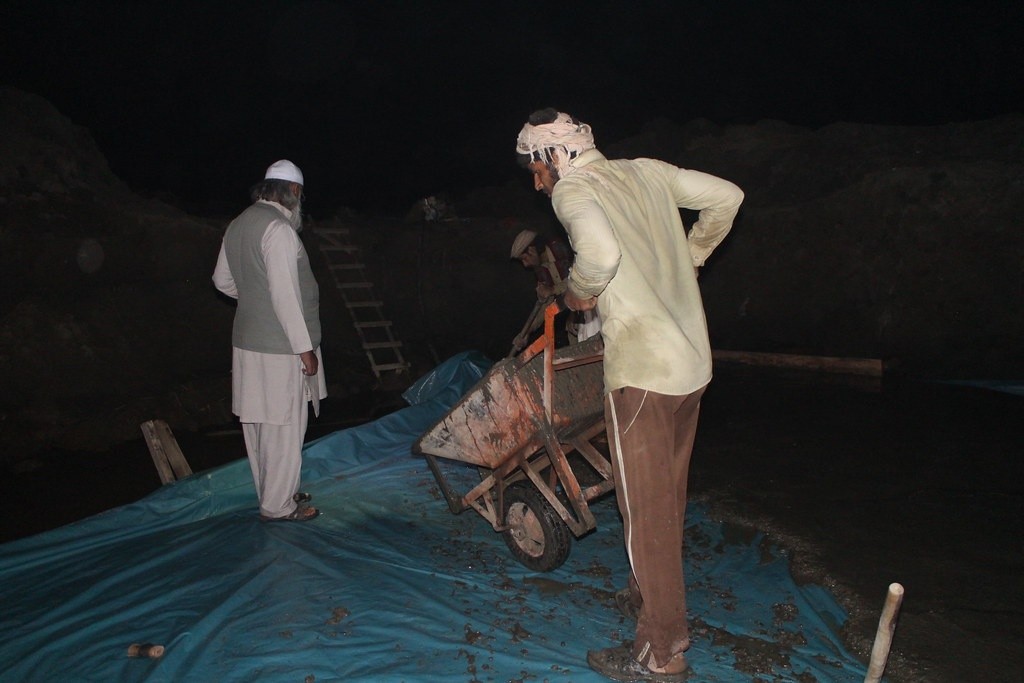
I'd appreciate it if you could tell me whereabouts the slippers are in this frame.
[587,639,688,682]
[614,587,641,623]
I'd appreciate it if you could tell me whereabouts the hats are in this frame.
[511,229,538,258]
[265,159,304,186]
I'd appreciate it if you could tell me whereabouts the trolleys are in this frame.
[411,278,616,573]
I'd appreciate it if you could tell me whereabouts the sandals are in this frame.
[294,492,312,503]
[278,504,320,521]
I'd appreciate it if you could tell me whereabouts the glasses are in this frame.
[299,187,306,202]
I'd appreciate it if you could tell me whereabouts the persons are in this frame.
[511,231,601,348]
[515,109,747,682]
[211,159,329,521]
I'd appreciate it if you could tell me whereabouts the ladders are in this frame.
[309,214,412,382]
